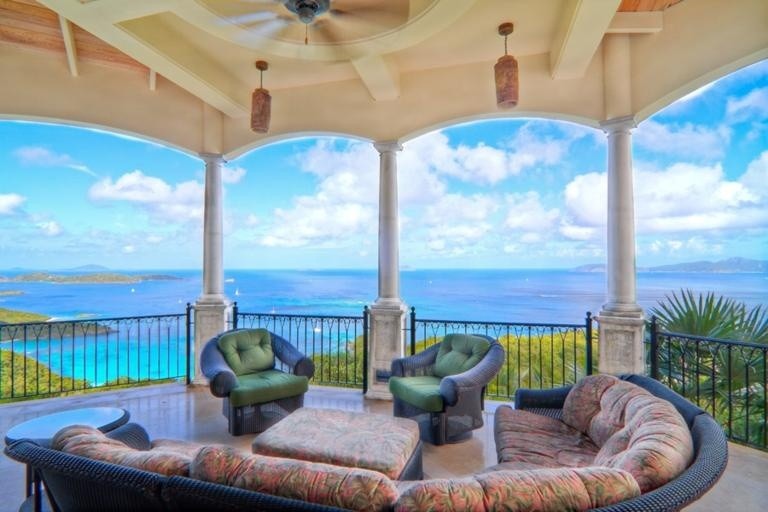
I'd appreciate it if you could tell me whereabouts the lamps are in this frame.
[285,0,330,45]
[250,61,271,134]
[494,23,520,107]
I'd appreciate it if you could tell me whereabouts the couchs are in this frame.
[4,373,728,511]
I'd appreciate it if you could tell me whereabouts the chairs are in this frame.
[201,328,315,435]
[389,334,505,444]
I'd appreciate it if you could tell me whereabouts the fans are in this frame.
[215,0,408,49]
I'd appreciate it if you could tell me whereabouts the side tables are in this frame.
[4,407,130,512]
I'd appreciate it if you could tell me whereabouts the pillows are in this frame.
[188,444,399,511]
[393,466,641,511]
[51,424,192,481]
[562,374,672,449]
[595,403,696,493]
